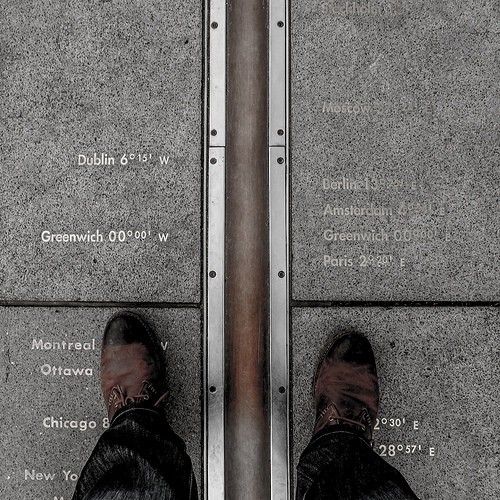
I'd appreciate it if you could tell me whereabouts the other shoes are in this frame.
[100,310,170,426]
[309,332,379,447]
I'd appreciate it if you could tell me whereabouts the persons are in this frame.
[73,310,420,500]
